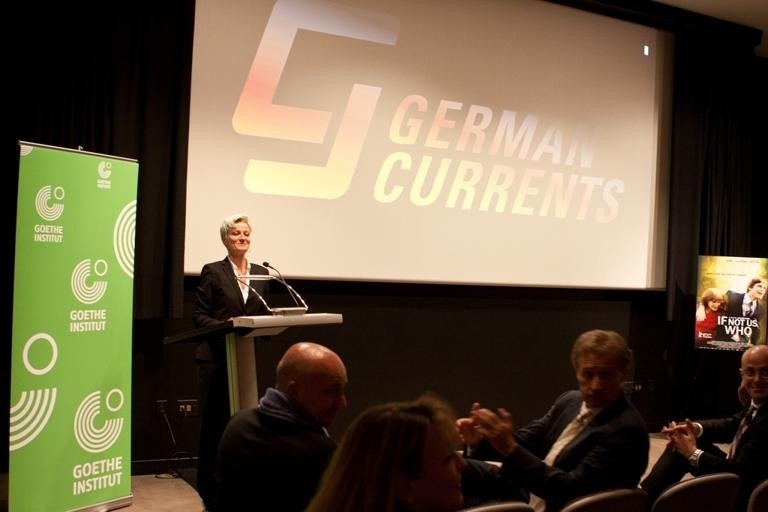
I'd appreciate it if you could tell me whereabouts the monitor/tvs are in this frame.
[694,254,768,354]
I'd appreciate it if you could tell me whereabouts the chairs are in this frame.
[652,472,741,512]
[457,501,536,512]
[747,479,768,512]
[559,487,650,512]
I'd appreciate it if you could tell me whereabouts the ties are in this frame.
[745,302,753,318]
[726,405,757,460]
[529,409,593,511]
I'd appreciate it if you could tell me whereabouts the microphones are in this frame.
[263,262,304,307]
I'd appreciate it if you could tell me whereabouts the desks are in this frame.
[106,473,206,512]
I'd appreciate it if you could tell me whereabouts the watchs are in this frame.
[688,449,704,467]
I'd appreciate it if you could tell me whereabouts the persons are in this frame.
[304,390,464,512]
[192,215,269,371]
[212,342,348,512]
[695,280,768,347]
[456,330,648,504]
[641,344,768,504]
